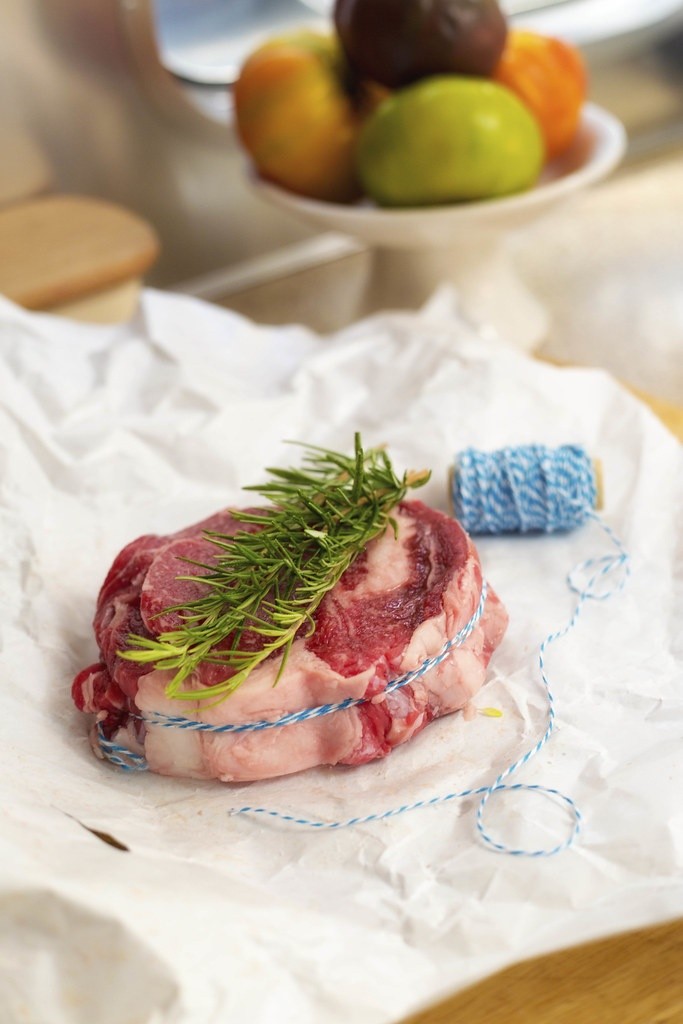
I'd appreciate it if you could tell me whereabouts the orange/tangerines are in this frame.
[234,2,591,212]
